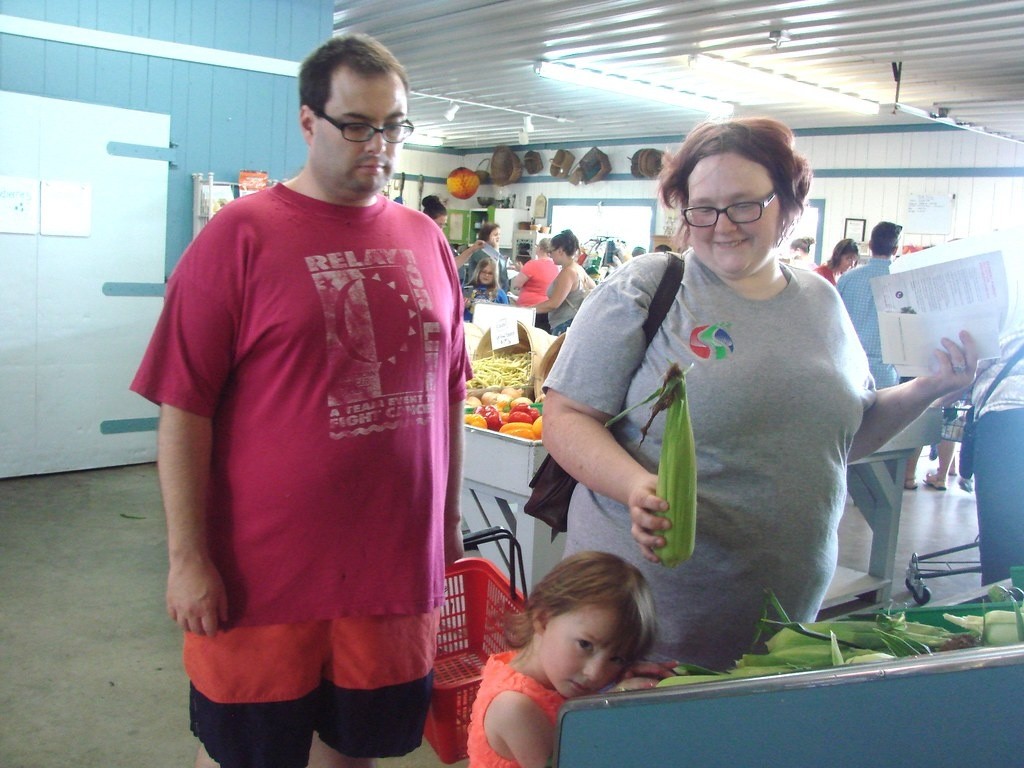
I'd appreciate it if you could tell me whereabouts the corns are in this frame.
[654,604,1023,691]
[649,362,698,566]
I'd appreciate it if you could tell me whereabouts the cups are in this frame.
[497,200,510,208]
[530,225,551,233]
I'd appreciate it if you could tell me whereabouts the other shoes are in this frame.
[929,449,938,461]
[937,467,958,476]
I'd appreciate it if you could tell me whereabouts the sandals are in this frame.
[904,476,918,490]
[923,471,946,490]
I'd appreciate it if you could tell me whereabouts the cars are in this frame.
[581,236,629,259]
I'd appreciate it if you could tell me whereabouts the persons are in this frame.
[130,33,468,768]
[789,221,1023,591]
[422,196,672,338]
[538,120,979,676]
[465,550,682,768]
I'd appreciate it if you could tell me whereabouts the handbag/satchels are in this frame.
[523,430,604,528]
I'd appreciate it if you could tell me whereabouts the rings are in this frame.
[952,365,967,373]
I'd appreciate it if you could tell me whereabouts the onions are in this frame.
[465,386,534,408]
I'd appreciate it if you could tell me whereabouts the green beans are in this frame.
[466,351,531,388]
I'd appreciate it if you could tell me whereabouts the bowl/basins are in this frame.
[477,197,494,207]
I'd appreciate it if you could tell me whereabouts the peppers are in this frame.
[465,400,544,440]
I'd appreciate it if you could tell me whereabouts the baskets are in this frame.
[423,525,530,764]
[940,399,973,442]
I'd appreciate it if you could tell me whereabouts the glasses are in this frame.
[545,247,559,255]
[682,190,778,227]
[312,106,414,143]
[480,271,495,276]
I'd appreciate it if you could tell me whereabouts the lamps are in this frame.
[518,128,529,145]
[523,116,534,133]
[443,104,460,122]
[533,62,734,117]
